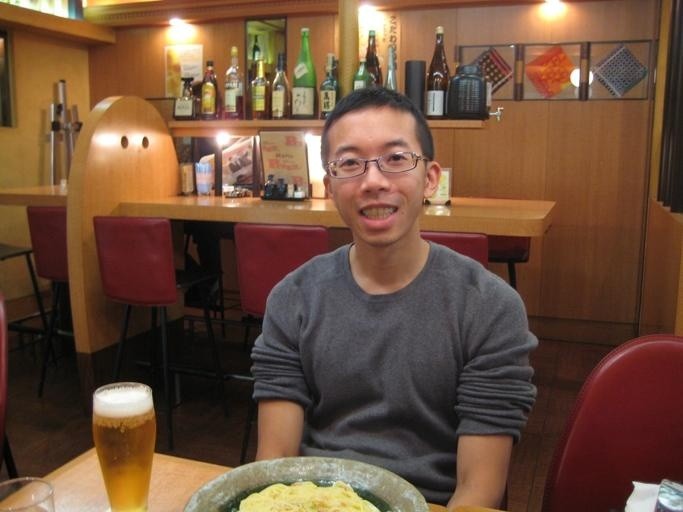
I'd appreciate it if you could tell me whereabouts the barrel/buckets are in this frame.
[445,64,490,121]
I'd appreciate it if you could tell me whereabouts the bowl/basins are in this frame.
[182,457,430,511]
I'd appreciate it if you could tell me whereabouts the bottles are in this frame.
[263,173,305,198]
[172,25,450,121]
[450,63,491,119]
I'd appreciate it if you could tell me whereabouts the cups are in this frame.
[89,382,157,511]
[0,476,53,512]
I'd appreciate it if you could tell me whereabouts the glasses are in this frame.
[324,150,429,180]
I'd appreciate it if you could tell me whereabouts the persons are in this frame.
[244,87,532,511]
[228,149,252,184]
